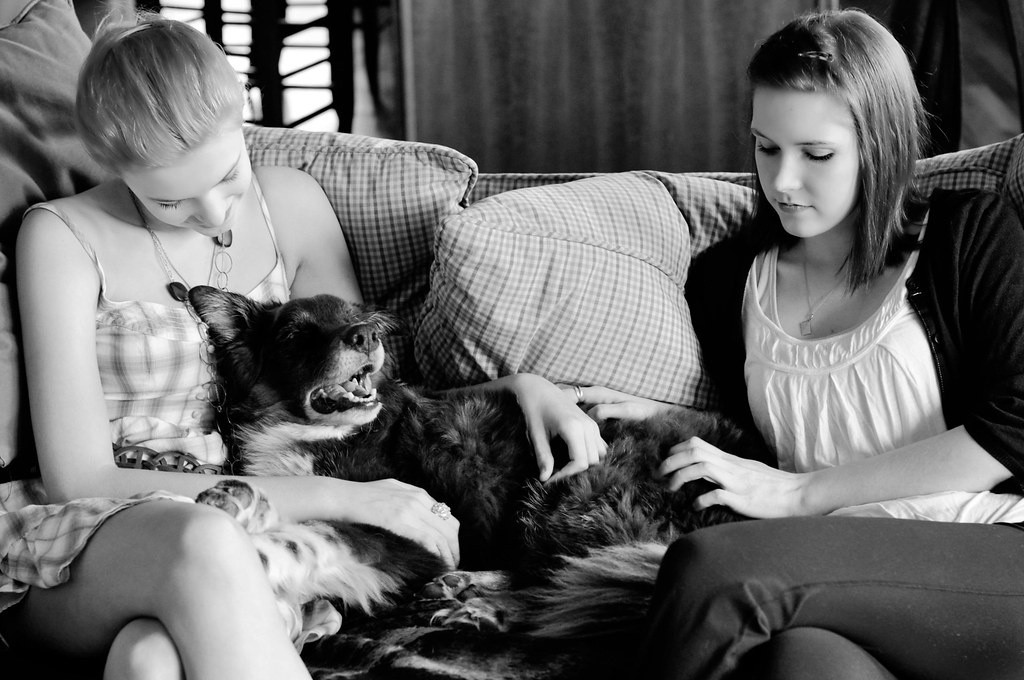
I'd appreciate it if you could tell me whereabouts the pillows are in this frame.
[1,0,769,417]
[234,118,478,391]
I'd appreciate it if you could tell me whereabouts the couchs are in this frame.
[2,171,1024,680]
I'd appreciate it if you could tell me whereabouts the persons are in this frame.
[553,5,1024,680]
[0,4,608,680]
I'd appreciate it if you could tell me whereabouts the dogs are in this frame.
[189,285,765,637]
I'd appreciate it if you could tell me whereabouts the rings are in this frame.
[432,502,451,520]
[575,385,585,401]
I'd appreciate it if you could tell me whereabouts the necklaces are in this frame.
[799,255,846,336]
[129,187,246,479]
